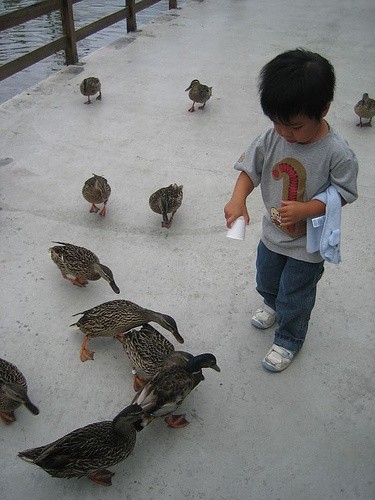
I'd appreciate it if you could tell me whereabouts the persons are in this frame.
[223,47,360,372]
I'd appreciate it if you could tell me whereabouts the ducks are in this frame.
[0,76,222,484]
[353,93,375,127]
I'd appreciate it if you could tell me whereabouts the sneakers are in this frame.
[261,343,297,371]
[251,302,278,330]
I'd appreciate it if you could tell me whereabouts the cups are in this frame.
[225,216,246,241]
